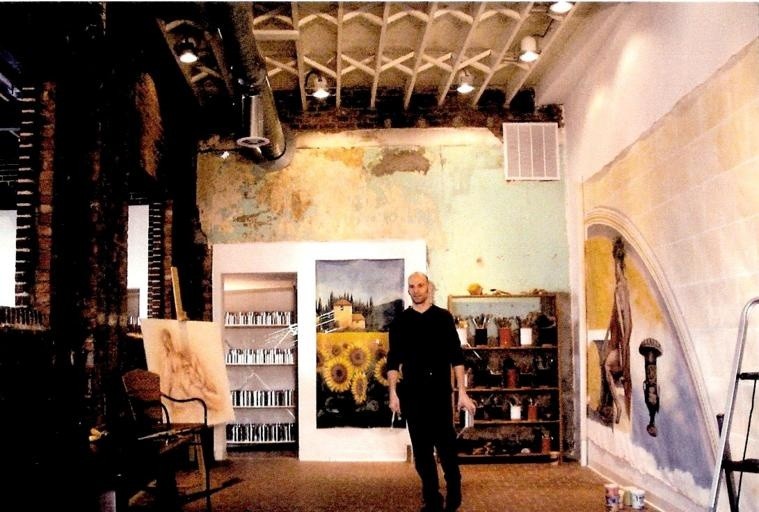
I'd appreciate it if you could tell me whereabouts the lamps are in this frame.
[519,36,539,62]
[313,72,331,99]
[178,33,198,64]
[456,68,475,94]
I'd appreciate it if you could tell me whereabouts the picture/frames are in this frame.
[316,258,404,429]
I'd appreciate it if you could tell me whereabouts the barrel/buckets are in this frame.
[603,482,624,512]
[630,489,645,510]
[623,486,637,507]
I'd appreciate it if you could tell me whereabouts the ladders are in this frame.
[706,296,759,512]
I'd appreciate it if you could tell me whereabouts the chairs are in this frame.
[121,369,211,498]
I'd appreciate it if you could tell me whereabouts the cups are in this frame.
[128,314,144,333]
[605,483,647,512]
[459,404,555,429]
[464,358,555,388]
[471,438,552,455]
[225,348,295,364]
[226,422,295,442]
[231,390,296,406]
[224,311,294,326]
[456,326,554,348]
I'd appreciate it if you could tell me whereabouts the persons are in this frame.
[596,236,637,427]
[385,267,479,512]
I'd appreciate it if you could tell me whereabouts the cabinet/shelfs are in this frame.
[448,293,565,465]
[221,272,300,459]
